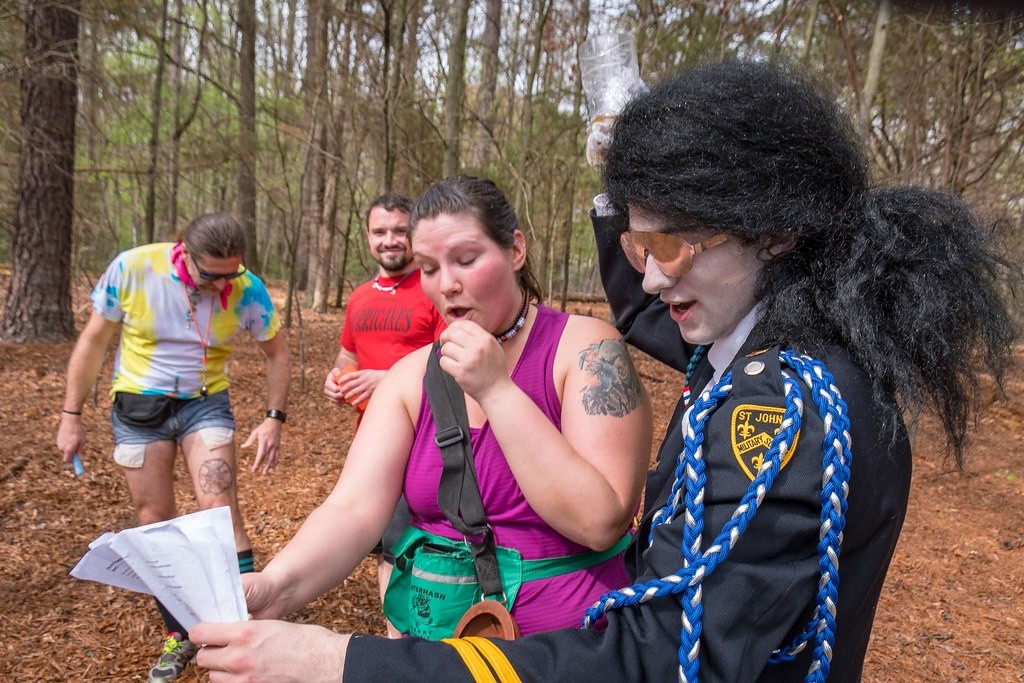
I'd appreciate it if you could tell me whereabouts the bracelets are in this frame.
[62,410,81,415]
[267,409,286,423]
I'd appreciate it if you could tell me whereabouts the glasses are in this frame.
[189,253,247,281]
[621,228,734,280]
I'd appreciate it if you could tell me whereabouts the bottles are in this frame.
[578,32,641,159]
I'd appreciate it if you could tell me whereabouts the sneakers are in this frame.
[148,633,202,683]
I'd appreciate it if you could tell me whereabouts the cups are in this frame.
[334,365,373,411]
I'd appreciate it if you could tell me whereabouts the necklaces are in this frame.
[374,267,416,291]
[494,287,529,344]
[182,281,215,403]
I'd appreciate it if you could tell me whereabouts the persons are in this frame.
[56,212,292,683]
[190,61,1018,683]
[239,175,653,641]
[323,191,451,639]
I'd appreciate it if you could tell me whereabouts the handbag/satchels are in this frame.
[383,529,524,642]
[113,391,199,427]
